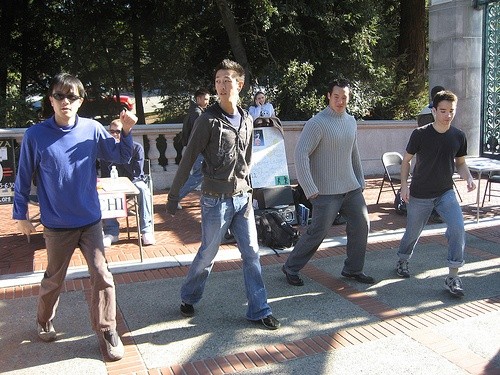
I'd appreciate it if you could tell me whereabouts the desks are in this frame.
[452,156,500,225]
[24,177,144,263]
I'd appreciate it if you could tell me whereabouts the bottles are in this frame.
[110,165,118,182]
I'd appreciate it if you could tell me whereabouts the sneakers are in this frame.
[395,259,411,278]
[248,314,281,329]
[176,202,183,210]
[179,300,194,316]
[444,274,464,296]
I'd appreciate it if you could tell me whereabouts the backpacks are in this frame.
[294,183,312,224]
[254,117,285,140]
[260,212,299,256]
[393,187,408,217]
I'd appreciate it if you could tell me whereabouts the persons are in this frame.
[101,118,155,246]
[166,59,282,330]
[281,78,376,286]
[176,89,210,210]
[248,91,276,123]
[13,73,138,361]
[418,86,446,128]
[395,90,476,297]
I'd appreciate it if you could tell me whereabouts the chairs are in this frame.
[482,154,500,207]
[375,151,403,205]
[125,158,153,243]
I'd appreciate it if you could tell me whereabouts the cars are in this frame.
[24,84,134,121]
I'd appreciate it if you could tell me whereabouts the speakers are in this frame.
[257,186,300,224]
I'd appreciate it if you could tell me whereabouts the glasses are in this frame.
[109,130,120,134]
[50,92,82,101]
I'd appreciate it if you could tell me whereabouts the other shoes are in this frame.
[340,269,373,284]
[37,319,58,343]
[103,234,118,247]
[283,265,304,286]
[103,328,125,360]
[142,232,153,245]
